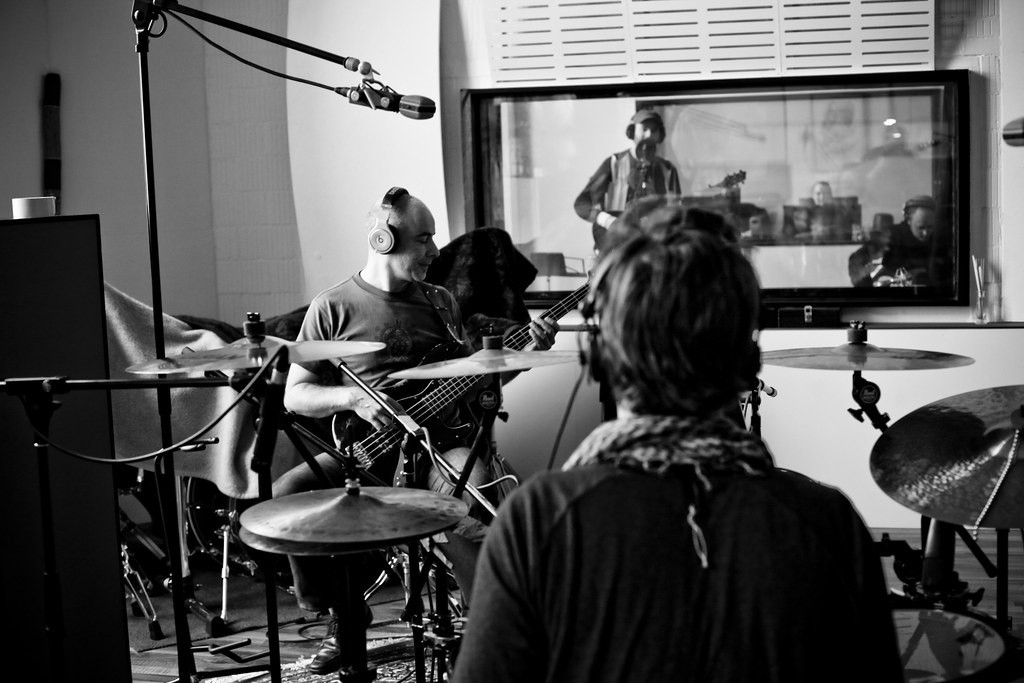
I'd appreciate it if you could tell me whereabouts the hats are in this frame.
[905,195,933,208]
[632,109,666,124]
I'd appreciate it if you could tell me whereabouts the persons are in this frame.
[574,109,682,254]
[450,203,907,683]
[848,196,939,287]
[284,188,561,674]
[741,182,852,245]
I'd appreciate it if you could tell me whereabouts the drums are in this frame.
[885,596,1017,683]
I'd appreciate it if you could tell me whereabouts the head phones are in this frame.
[368,186,408,254]
[626,122,666,143]
[576,205,764,386]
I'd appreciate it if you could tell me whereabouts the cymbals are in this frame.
[124,335,386,374]
[760,343,976,370]
[869,384,1024,528]
[387,347,586,379]
[238,487,469,556]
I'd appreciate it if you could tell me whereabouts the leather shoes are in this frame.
[308,605,374,673]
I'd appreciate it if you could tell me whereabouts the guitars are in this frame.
[592,170,747,252]
[331,265,600,487]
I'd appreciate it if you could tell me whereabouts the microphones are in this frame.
[334,87,436,120]
[250,349,291,474]
[921,518,955,599]
